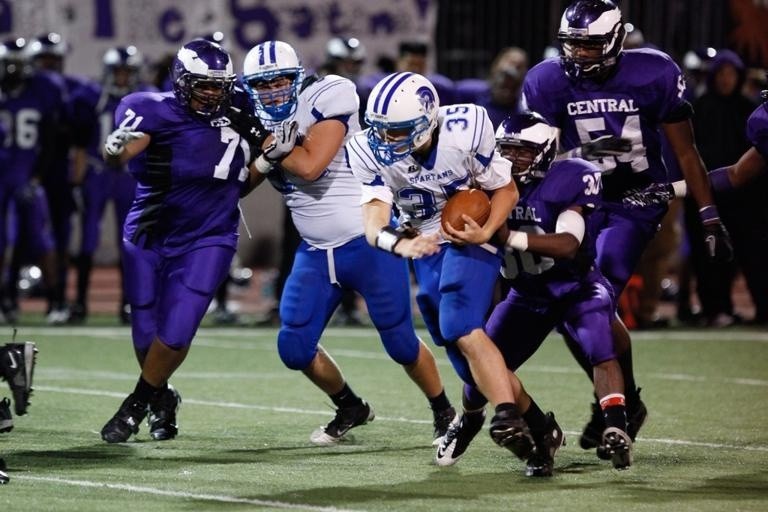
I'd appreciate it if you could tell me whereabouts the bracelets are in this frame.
[670,180,688,199]
[375,226,404,256]
[505,230,529,253]
[254,153,275,175]
[554,209,587,246]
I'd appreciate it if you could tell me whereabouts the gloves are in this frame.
[622,180,678,211]
[256,120,304,164]
[572,133,635,161]
[698,211,733,259]
[104,126,149,160]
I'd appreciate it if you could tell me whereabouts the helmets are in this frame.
[0,36,44,61]
[37,33,67,58]
[241,40,305,131]
[169,38,238,118]
[363,71,442,168]
[557,0,627,82]
[104,45,144,71]
[492,111,563,187]
[326,34,365,64]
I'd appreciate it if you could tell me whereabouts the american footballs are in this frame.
[441,189,493,232]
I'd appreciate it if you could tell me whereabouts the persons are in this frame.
[655,66,703,324]
[100,39,300,441]
[221,38,456,447]
[474,70,523,123]
[519,0,737,462]
[68,43,159,326]
[368,51,396,78]
[433,109,634,471]
[739,66,767,104]
[0,341,40,433]
[346,70,567,477]
[454,45,529,105]
[395,40,458,106]
[144,53,178,92]
[696,47,767,331]
[0,35,73,327]
[10,31,80,324]
[0,456,11,484]
[619,88,767,213]
[321,37,385,109]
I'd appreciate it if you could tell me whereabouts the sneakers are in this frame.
[146,383,182,442]
[102,395,147,444]
[578,387,647,449]
[0,395,14,434]
[598,401,633,471]
[431,405,457,444]
[434,406,489,467]
[2,340,38,418]
[489,411,538,462]
[309,396,378,448]
[525,411,569,478]
